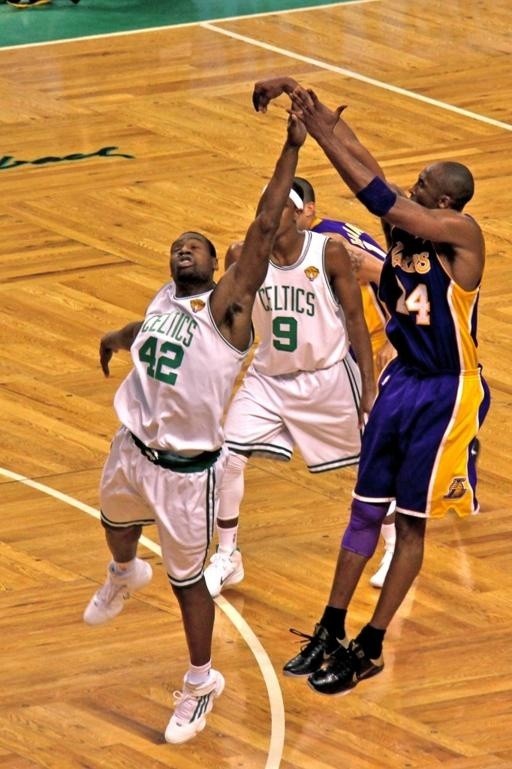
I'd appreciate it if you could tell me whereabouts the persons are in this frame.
[289,177,400,400]
[202,178,396,597]
[82,103,307,748]
[250,74,491,699]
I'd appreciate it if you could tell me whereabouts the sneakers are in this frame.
[162,666,226,745]
[281,621,351,677]
[81,556,153,625]
[307,639,385,697]
[369,543,397,588]
[202,542,244,600]
